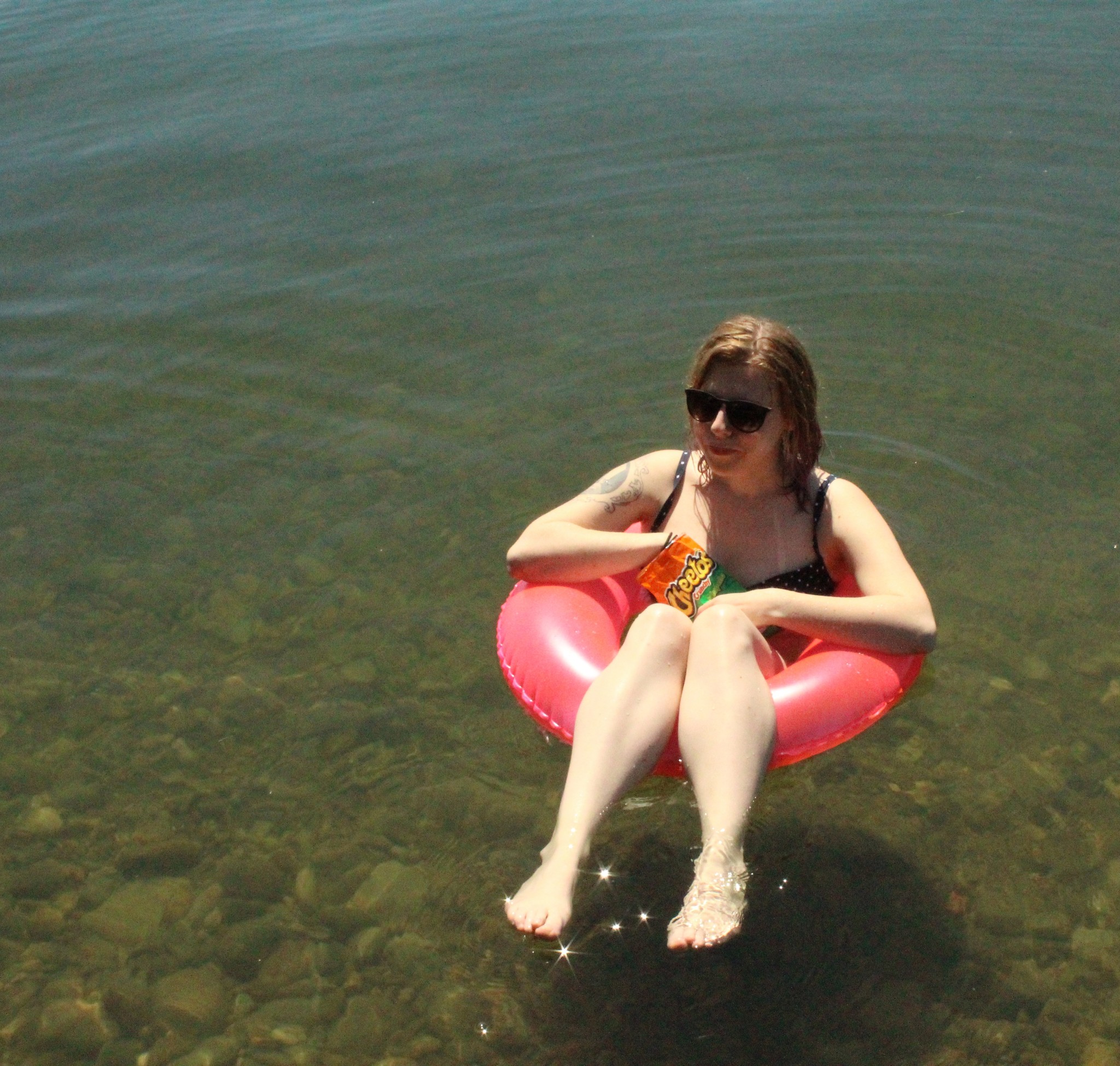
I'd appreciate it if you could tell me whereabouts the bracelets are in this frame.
[663,532,672,549]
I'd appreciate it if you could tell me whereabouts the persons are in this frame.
[504,314,937,860]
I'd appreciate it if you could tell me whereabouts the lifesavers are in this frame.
[495,517,924,777]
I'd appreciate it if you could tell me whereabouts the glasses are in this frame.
[685,386,781,434]
